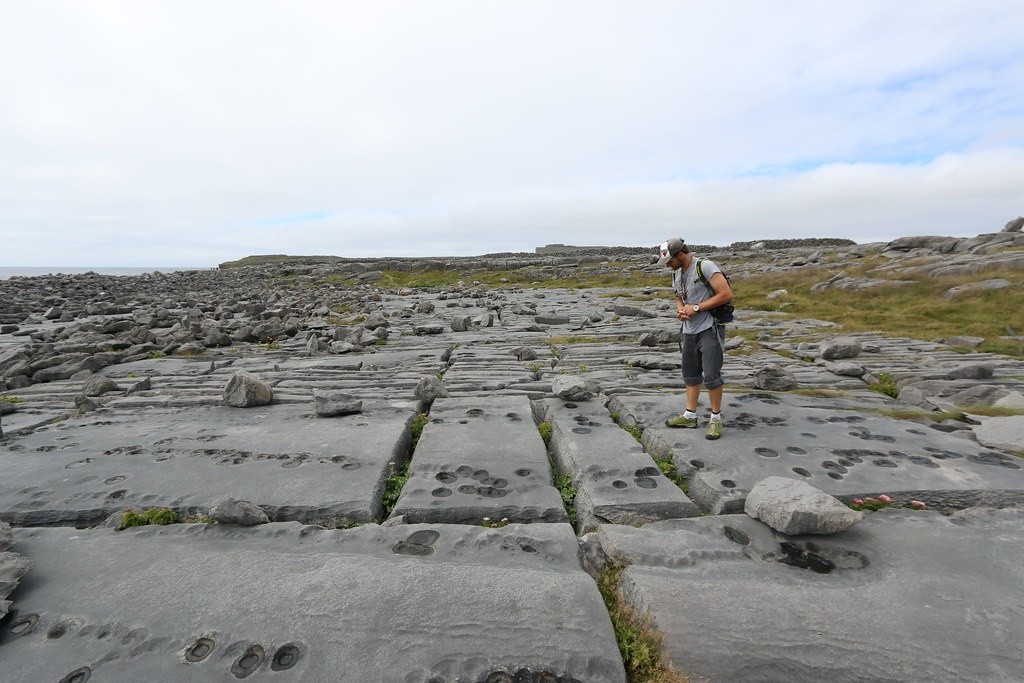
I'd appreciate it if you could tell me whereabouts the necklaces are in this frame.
[683,256,692,271]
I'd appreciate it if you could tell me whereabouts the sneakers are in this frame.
[664,415,698,427]
[704,420,722,440]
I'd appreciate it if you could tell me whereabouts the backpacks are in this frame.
[672,257,734,325]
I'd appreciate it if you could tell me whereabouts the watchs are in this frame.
[693,304,700,314]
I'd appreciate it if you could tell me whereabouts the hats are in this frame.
[656,238,684,266]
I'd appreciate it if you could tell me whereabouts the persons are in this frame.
[657,238,734,441]
